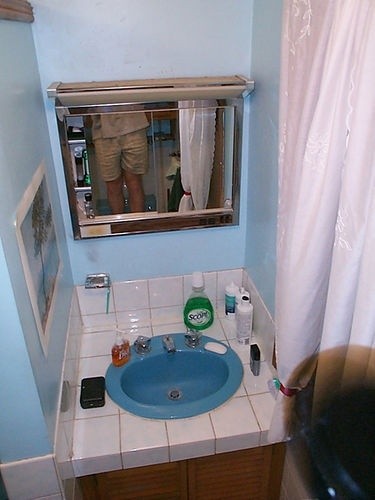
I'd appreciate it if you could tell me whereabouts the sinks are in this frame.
[118,350,229,408]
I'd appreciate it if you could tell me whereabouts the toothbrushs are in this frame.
[103,276,111,314]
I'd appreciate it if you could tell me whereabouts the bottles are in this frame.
[74,147,83,186]
[84,192,94,218]
[81,150,93,186]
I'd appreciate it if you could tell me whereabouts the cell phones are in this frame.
[80,375,106,409]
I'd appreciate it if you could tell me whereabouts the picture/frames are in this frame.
[13,159,65,357]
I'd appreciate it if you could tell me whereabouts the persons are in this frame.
[87,104,151,216]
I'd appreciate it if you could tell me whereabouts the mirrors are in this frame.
[55,98,244,240]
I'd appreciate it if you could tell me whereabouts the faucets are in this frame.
[161,335,176,356]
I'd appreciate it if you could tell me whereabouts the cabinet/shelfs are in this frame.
[61,107,179,218]
